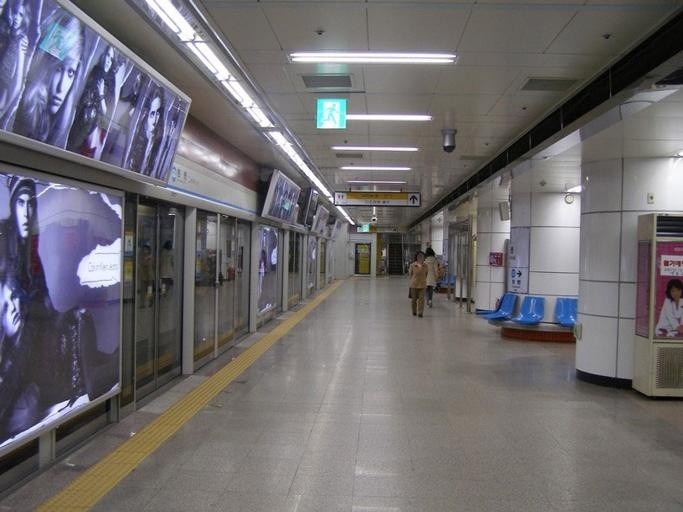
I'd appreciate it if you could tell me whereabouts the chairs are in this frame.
[437,273,456,294]
[476,293,578,327]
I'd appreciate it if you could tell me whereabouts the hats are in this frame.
[6,176,38,212]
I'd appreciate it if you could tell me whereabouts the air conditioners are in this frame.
[632,211,683,399]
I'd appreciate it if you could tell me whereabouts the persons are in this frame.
[0,173,55,442]
[265,229,277,306]
[654,278,682,338]
[138,244,154,309]
[258,249,275,308]
[423,247,441,308]
[159,239,174,296]
[1,265,119,425]
[433,262,444,292]
[0,0,189,181]
[407,251,428,317]
[236,246,242,279]
[267,176,298,221]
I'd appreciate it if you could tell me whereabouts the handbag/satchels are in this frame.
[408,287,411,298]
[432,258,445,280]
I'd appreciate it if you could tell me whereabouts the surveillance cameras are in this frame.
[371,216,377,223]
[439,135,457,153]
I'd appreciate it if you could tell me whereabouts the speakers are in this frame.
[499,202,509,220]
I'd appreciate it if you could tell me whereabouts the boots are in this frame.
[412,313,423,317]
[427,300,432,308]
